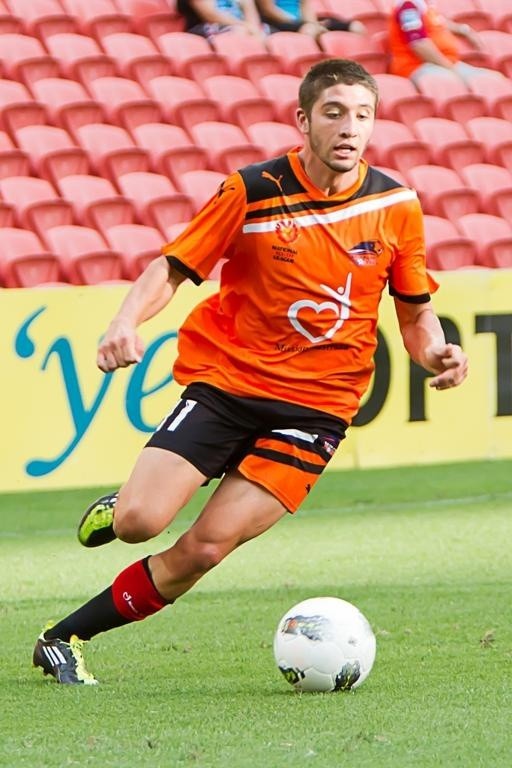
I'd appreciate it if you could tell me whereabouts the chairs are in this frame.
[0,1,512,288]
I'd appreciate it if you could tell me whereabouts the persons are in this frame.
[255,0,324,37]
[33,58,469,685]
[178,0,245,39]
[387,0,459,78]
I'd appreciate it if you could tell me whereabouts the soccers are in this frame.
[275,596,375,691]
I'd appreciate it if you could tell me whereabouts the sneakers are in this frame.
[78,489,122,548]
[32,619,99,685]
